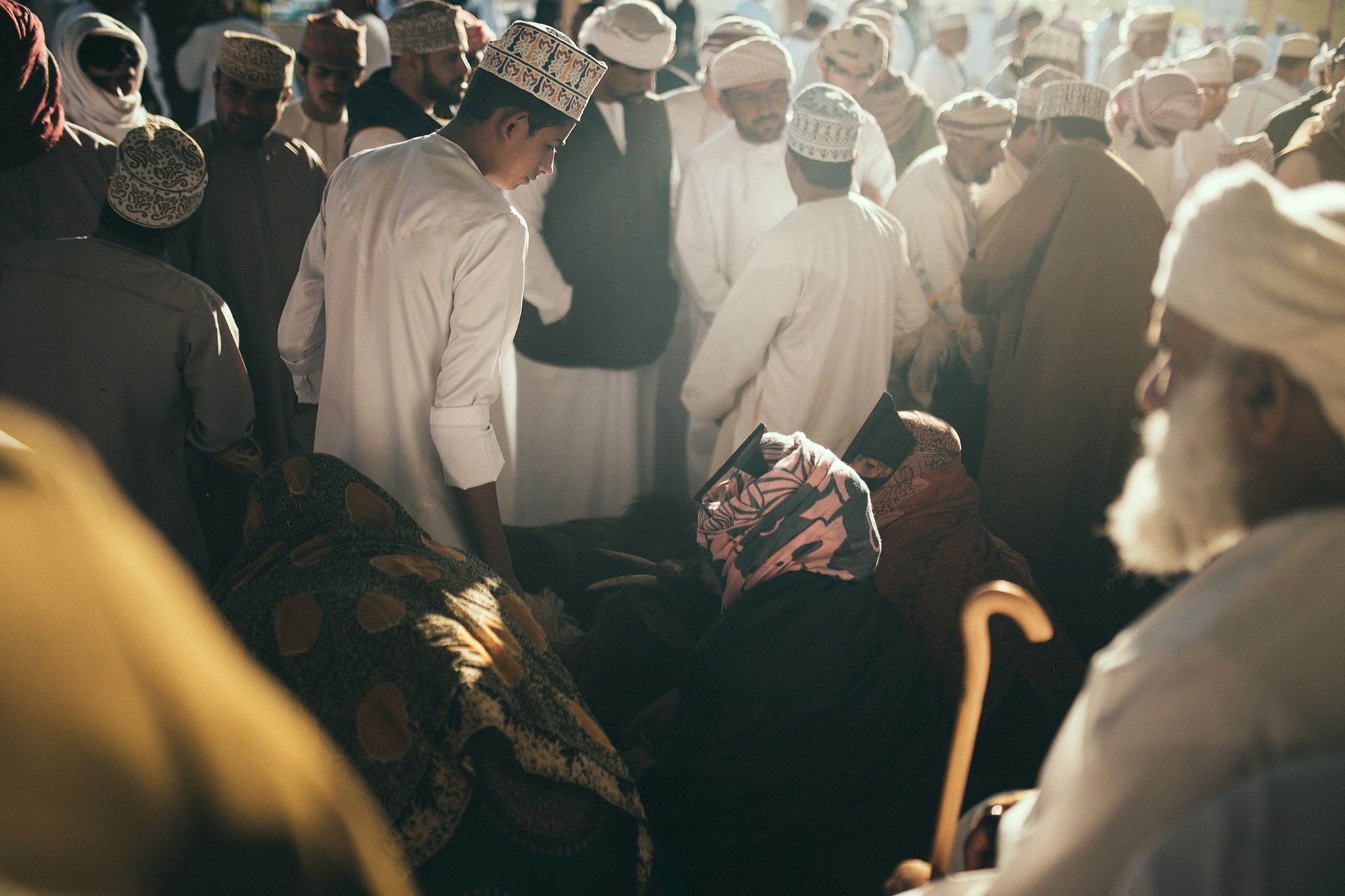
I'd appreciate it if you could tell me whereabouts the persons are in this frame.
[896,154,1345,896]
[0,0,1345,896]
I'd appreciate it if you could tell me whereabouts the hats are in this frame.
[462,10,497,51]
[1013,65,1081,120]
[822,16,887,72]
[1171,42,1233,84]
[1149,161,1345,438]
[386,1,469,54]
[1220,132,1276,175]
[300,9,367,69]
[1131,68,1209,145]
[216,29,295,90]
[1024,28,1080,63]
[935,91,1018,139]
[578,0,677,71]
[789,82,863,162]
[710,36,796,90]
[1278,32,1319,58]
[929,7,967,32]
[701,17,782,53]
[107,123,209,229]
[1228,35,1271,70]
[1037,79,1110,124]
[1127,8,1173,33]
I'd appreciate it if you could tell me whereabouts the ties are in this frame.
[478,19,609,121]
